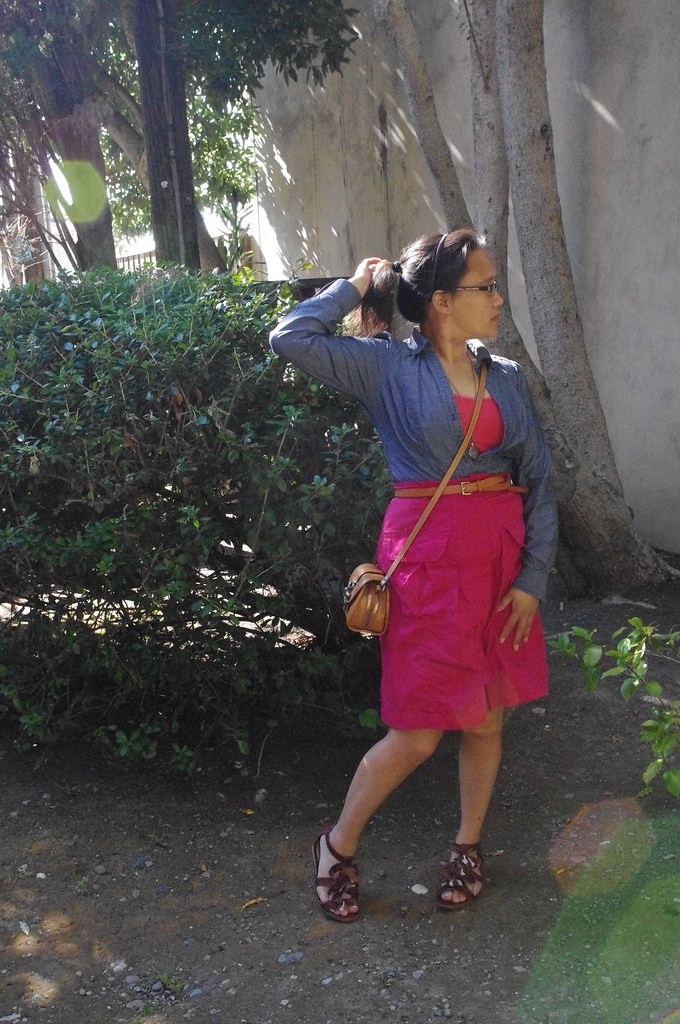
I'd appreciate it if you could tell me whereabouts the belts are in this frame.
[394,475,529,498]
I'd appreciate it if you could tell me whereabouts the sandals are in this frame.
[311,821,361,922]
[436,841,483,910]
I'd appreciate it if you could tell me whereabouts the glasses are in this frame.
[447,281,498,293]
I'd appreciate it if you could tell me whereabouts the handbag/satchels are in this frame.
[342,563,390,638]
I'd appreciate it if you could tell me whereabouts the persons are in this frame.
[267,228,556,922]
